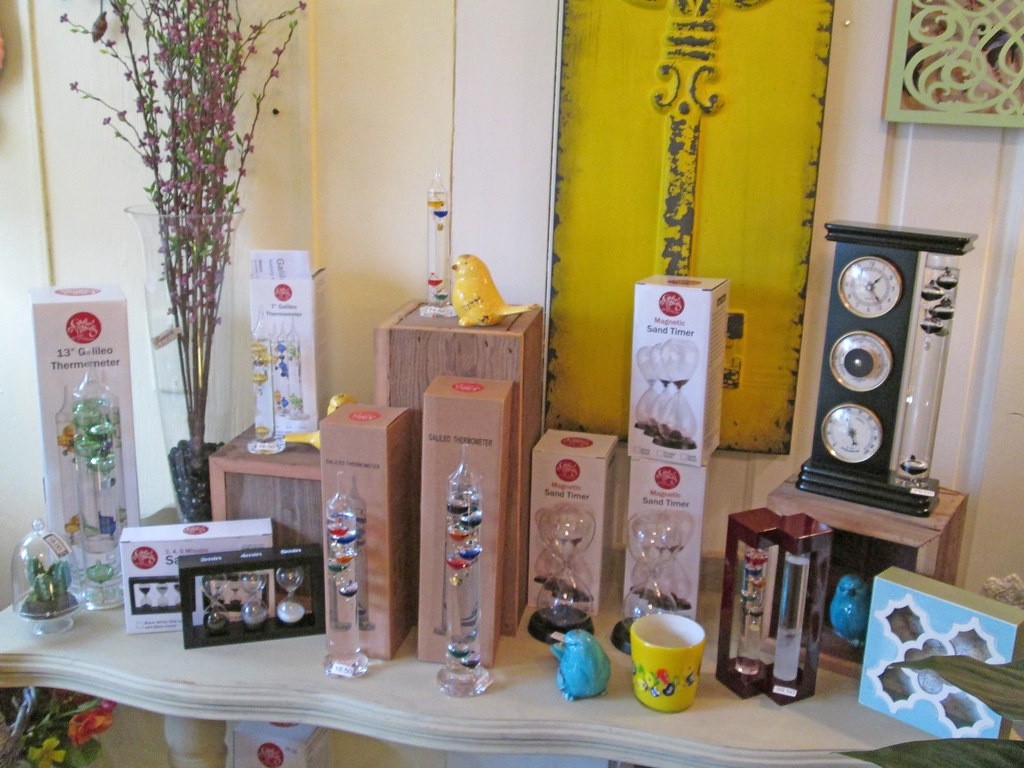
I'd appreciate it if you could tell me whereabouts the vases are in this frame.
[127,204,244,526]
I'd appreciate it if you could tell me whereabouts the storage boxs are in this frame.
[624,455,712,620]
[374,300,544,635]
[320,403,415,659]
[210,424,323,555]
[234,720,330,768]
[528,429,620,616]
[858,565,1024,740]
[178,543,325,649]
[628,275,730,468]
[249,246,328,441]
[442,750,620,768]
[419,375,514,670]
[121,517,273,634]
[30,280,142,596]
[767,466,970,679]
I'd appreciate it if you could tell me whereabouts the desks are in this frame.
[0,584,1012,768]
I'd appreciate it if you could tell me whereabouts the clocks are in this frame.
[796,219,979,517]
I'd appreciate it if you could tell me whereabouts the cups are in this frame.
[629,615,706,712]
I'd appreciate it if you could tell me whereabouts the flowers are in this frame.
[61,0,309,465]
[0,686,114,768]
